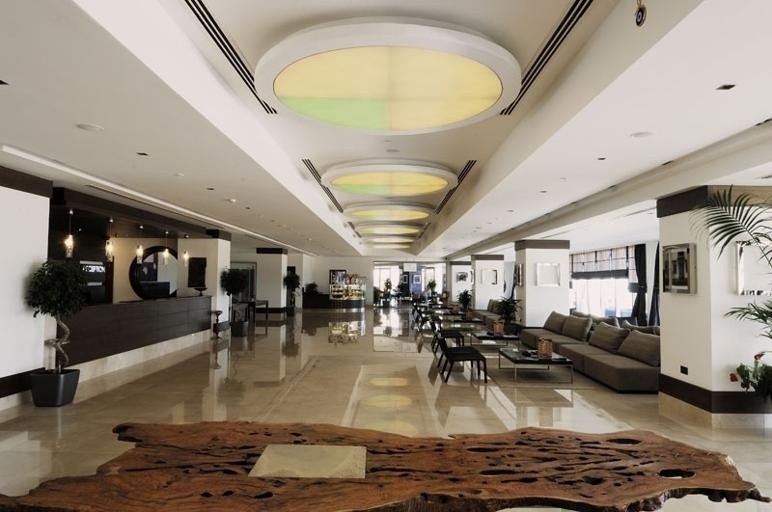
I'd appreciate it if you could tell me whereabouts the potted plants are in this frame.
[382,279,393,307]
[492,296,522,337]
[219,268,252,338]
[458,290,474,322]
[284,271,300,316]
[428,281,437,299]
[21,258,91,407]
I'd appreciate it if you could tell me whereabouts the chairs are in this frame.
[409,294,488,385]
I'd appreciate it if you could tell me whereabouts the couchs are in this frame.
[470,298,504,326]
[519,309,660,395]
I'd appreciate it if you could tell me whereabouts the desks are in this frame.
[232,299,269,340]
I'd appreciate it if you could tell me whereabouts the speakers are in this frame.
[187,257,207,287]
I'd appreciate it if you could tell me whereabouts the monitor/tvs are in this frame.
[80,264,106,288]
[140,281,170,300]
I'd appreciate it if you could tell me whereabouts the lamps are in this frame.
[321,159,459,198]
[62,211,191,268]
[253,16,526,136]
[342,200,436,222]
[354,223,426,249]
